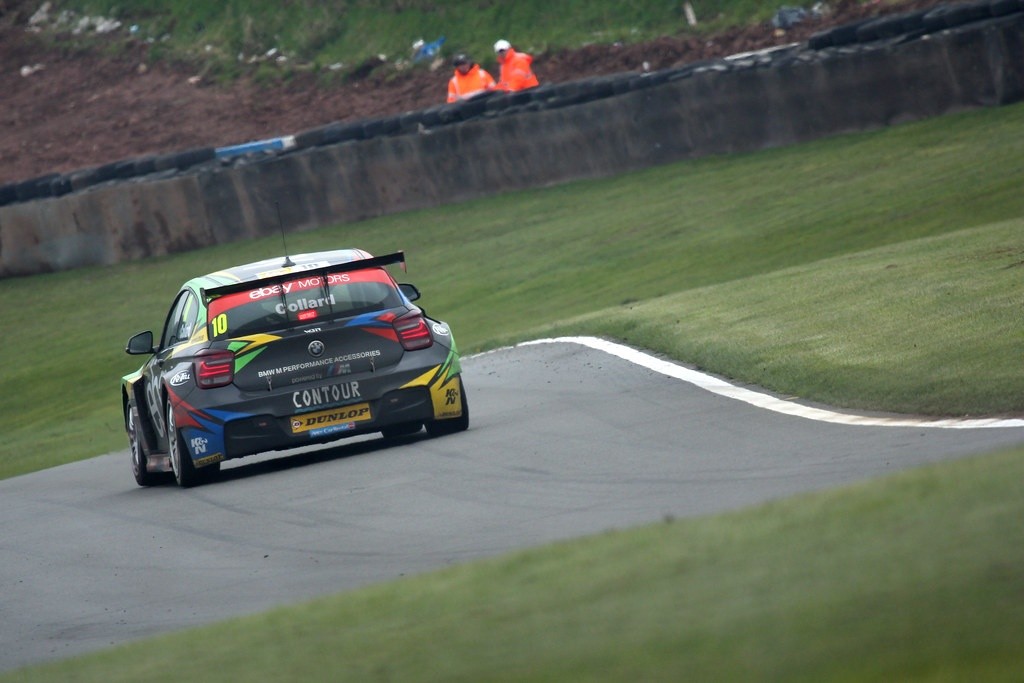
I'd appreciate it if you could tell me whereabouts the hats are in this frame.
[494,39,510,52]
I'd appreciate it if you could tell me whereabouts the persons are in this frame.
[491,39,539,93]
[446,54,496,103]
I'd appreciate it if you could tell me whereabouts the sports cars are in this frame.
[120,246,471,486]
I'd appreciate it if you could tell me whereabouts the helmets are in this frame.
[452,52,468,66]
